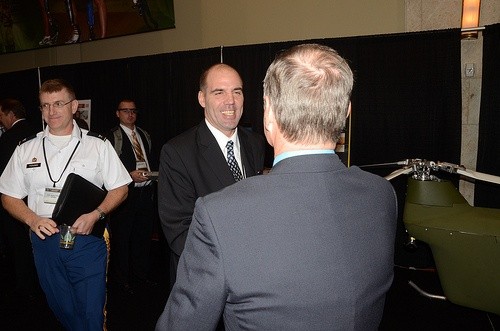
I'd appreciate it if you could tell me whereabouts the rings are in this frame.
[38,225,41,229]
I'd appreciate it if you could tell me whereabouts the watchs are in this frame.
[96,208,105,219]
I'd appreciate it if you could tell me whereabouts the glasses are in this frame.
[39,98,75,112]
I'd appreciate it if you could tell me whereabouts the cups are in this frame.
[59,225,77,250]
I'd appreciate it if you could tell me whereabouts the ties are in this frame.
[226,140,243,182]
[131,130,150,182]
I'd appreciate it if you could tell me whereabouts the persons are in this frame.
[0,99,37,264]
[155,43,397,331]
[158,64,271,282]
[102,99,161,294]
[0,79,132,331]
